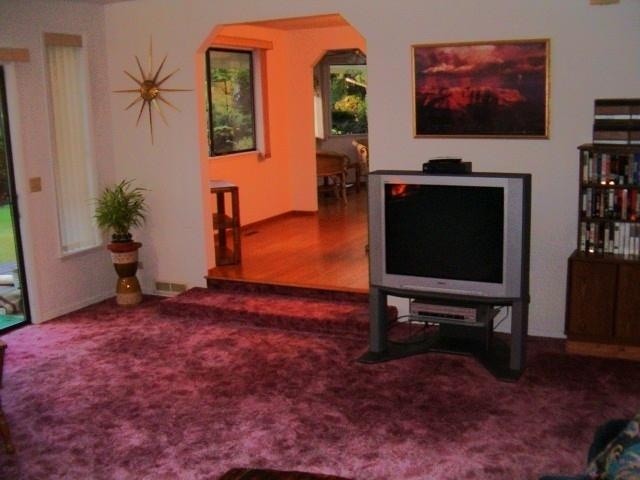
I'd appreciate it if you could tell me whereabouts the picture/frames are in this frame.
[409,38,551,141]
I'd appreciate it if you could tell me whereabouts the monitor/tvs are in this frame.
[365,169,532,301]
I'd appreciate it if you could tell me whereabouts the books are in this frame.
[580,148,639,256]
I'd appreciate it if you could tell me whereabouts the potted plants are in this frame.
[86,176,153,305]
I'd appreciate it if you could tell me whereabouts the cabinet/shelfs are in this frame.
[357,284,530,383]
[564,143,640,361]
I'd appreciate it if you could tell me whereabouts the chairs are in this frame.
[316,150,350,205]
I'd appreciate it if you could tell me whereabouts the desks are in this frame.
[210,180,241,266]
